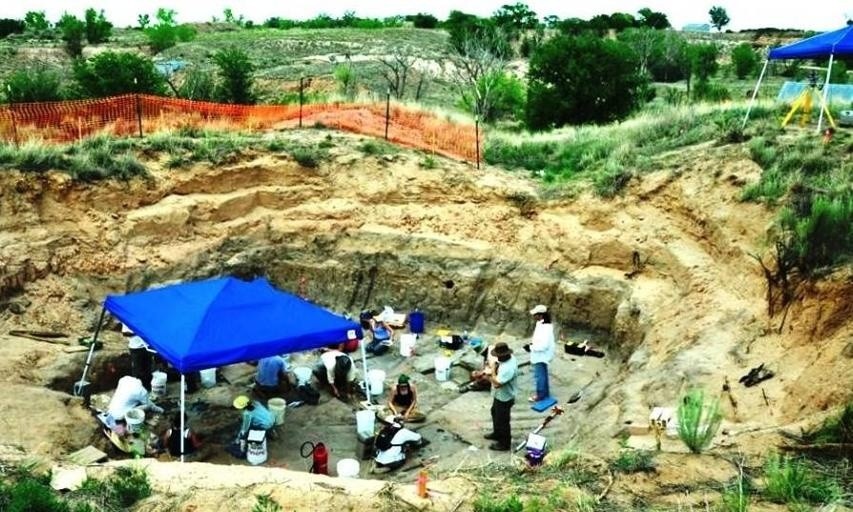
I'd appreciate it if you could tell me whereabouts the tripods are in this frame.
[779,86,839,132]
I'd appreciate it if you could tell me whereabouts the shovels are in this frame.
[569,371,601,403]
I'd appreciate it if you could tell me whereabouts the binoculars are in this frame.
[781,70,836,132]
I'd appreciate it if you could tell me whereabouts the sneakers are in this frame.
[484,433,498,440]
[491,443,511,451]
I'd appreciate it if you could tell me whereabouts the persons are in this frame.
[457,337,500,393]
[473,341,517,450]
[369,416,422,474]
[360,310,395,355]
[104,317,359,458]
[523,303,554,405]
[376,374,426,425]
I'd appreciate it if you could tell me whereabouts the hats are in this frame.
[530,304,546,315]
[399,375,410,384]
[491,342,513,356]
[233,396,250,410]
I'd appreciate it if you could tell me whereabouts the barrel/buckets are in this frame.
[269,398,286,425]
[400,334,416,356]
[151,381,166,398]
[369,369,385,395]
[336,457,360,477]
[435,357,451,381]
[356,410,375,439]
[200,369,216,388]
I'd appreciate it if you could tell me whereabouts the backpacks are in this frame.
[168,428,186,457]
[375,426,403,451]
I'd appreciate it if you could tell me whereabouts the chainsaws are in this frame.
[64,336,103,353]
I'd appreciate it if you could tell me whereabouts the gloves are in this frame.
[523,344,531,352]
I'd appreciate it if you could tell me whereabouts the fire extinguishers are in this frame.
[313,442,328,474]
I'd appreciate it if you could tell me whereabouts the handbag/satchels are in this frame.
[360,308,372,330]
[441,334,463,349]
